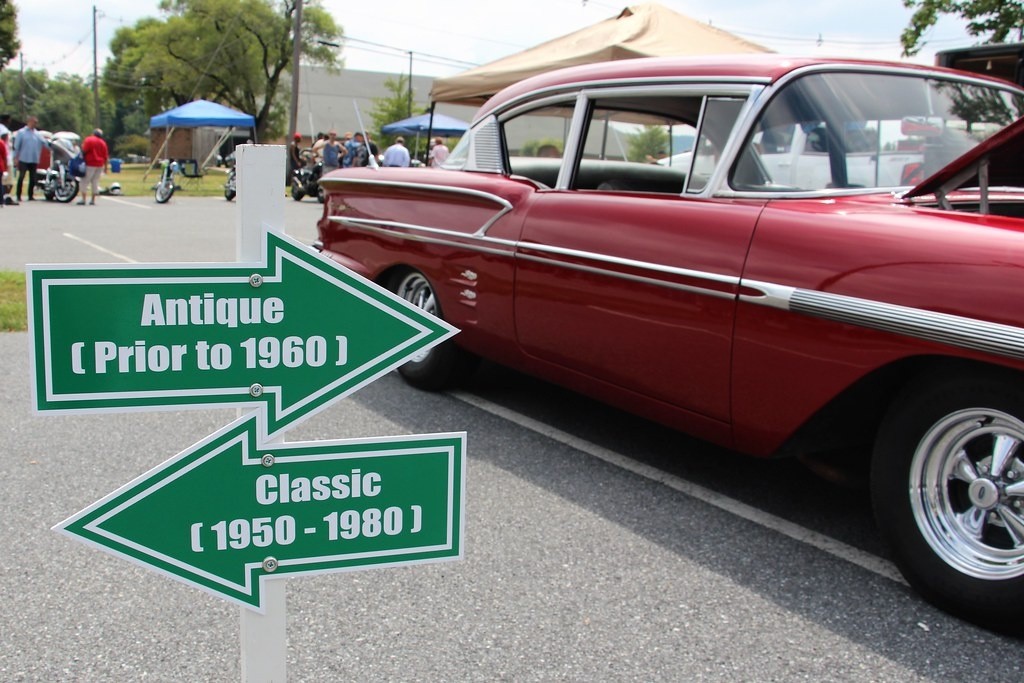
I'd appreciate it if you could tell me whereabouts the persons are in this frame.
[342,130,379,168]
[0,134,12,205]
[311,130,348,200]
[646,148,669,166]
[383,136,411,168]
[58,138,82,160]
[12,116,52,202]
[423,135,448,168]
[289,134,306,182]
[75,128,109,205]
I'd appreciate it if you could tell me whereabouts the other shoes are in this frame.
[5,197,19,205]
[17,198,21,201]
[89,201,95,204]
[0,199,5,204]
[29,197,34,200]
[77,202,85,205]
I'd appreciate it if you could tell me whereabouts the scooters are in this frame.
[291,163,325,200]
[224,166,237,200]
[151,160,180,203]
[33,142,81,203]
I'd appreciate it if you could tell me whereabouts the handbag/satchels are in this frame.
[68,151,86,177]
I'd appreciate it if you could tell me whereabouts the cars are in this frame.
[646,118,981,189]
[315,54,1024,638]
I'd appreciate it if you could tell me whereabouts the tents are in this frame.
[425,1,779,167]
[149,100,256,180]
[381,111,471,169]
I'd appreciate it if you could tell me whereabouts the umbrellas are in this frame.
[0,123,10,137]
[37,128,54,139]
[50,131,81,140]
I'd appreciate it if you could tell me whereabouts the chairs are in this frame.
[177,158,204,191]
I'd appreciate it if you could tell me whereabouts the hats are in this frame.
[93,128,102,137]
[344,132,352,139]
[395,136,404,144]
[294,133,302,139]
[429,139,436,146]
